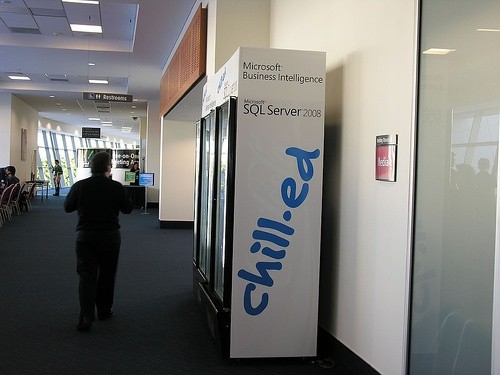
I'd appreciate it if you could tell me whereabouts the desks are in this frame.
[26,181,51,203]
[124,185,146,210]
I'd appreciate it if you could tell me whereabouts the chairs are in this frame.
[0,183,37,228]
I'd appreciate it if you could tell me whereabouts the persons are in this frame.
[50,160,63,197]
[0,166,20,201]
[64,152,133,331]
[130,162,140,186]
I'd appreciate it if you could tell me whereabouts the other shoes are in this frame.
[52,193,59,196]
[77,317,93,332]
[98,310,113,321]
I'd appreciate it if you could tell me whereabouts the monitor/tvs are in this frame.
[139,173,154,186]
